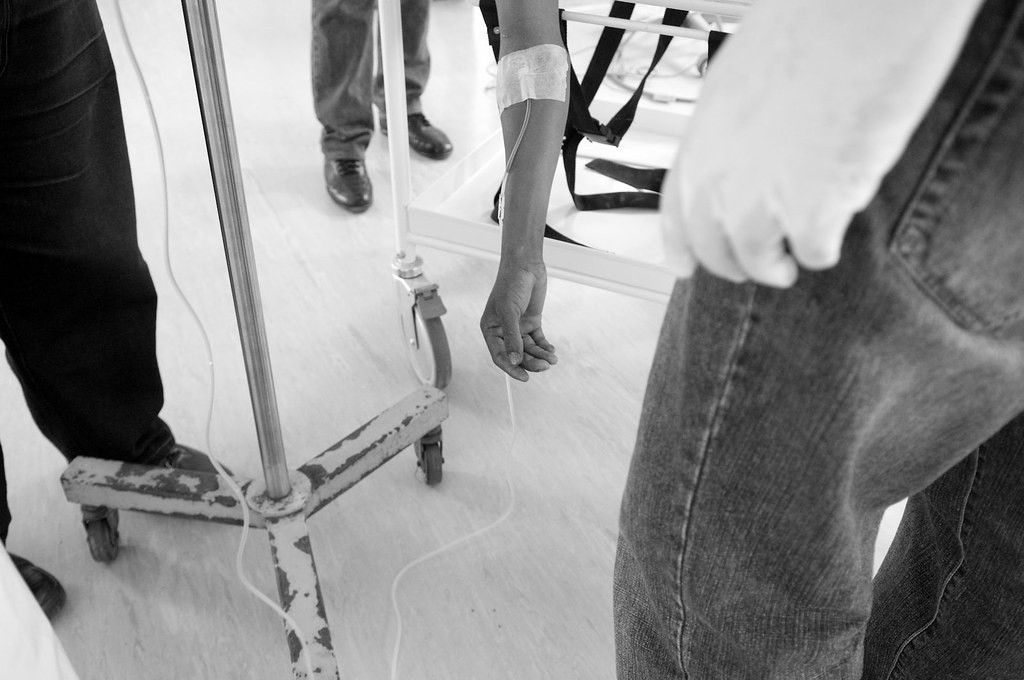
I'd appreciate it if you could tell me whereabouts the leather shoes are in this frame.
[380,112,454,159]
[324,157,372,213]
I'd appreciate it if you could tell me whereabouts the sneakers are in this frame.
[7,551,67,617]
[155,443,233,477]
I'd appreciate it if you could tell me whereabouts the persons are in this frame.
[613,0,1024,680]
[480,0,571,382]
[0,0,240,621]
[311,0,452,213]
